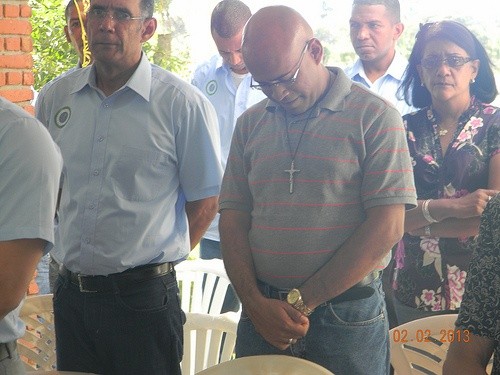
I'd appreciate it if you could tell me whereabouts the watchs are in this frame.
[287,288,315,317]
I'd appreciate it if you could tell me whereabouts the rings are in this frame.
[288,338,292,344]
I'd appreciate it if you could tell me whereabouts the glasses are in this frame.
[248,41,310,91]
[421,55,474,69]
[86,8,147,25]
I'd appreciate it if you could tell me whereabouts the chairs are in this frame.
[17,293,62,372]
[172,258,242,375]
[384,312,495,375]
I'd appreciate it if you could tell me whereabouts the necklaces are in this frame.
[438,124,452,136]
[283,105,315,195]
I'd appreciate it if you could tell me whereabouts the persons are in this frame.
[0,97,64,375]
[342,0,424,117]
[218,5,419,375]
[190,0,268,365]
[380,21,500,330]
[442,192,500,375]
[34,0,224,375]
[48,0,90,293]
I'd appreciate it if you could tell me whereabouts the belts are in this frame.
[258,269,383,306]
[57,261,176,293]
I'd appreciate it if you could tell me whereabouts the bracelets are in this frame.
[422,199,439,224]
[425,225,431,238]
